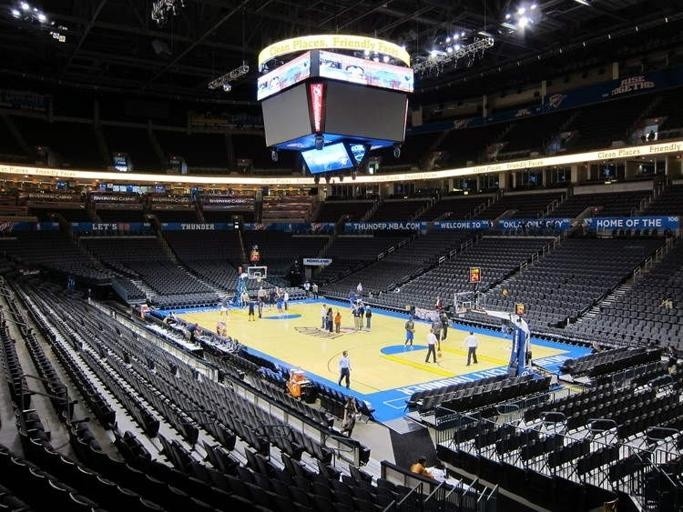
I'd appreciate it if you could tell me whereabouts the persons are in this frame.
[466,331,478,365]
[339,351,351,389]
[425,296,449,363]
[312,282,319,300]
[304,281,310,299]
[405,316,415,350]
[321,281,371,333]
[241,286,290,322]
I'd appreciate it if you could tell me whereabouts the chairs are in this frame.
[1,186,682,512]
